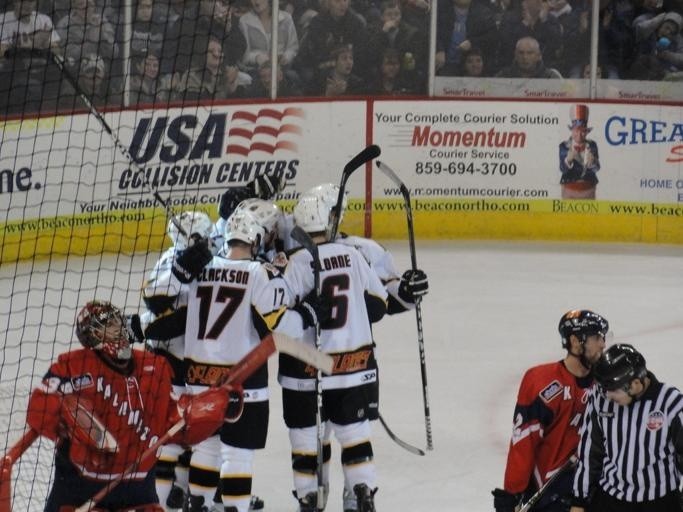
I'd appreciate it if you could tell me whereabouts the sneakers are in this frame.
[167,483,379,512]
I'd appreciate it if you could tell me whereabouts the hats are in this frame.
[567,105,593,133]
[664,11,682,33]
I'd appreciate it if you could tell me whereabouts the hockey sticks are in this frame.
[290,224,328,512]
[74,330,335,512]
[4,46,194,245]
[377,414,425,457]
[376,161,437,451]
[331,144,380,244]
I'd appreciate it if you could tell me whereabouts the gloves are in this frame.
[292,291,326,330]
[166,387,227,444]
[170,242,213,283]
[491,487,524,512]
[247,177,279,199]
[398,268,429,304]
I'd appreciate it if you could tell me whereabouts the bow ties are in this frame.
[574,143,585,151]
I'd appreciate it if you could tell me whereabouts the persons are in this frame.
[124,172,429,512]
[0,0,683,113]
[559,127,601,199]
[491,309,609,511]
[569,342,683,511]
[26,298,230,511]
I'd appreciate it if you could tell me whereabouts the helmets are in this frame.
[593,343,646,391]
[76,301,118,348]
[558,310,609,344]
[168,210,211,252]
[221,182,348,246]
